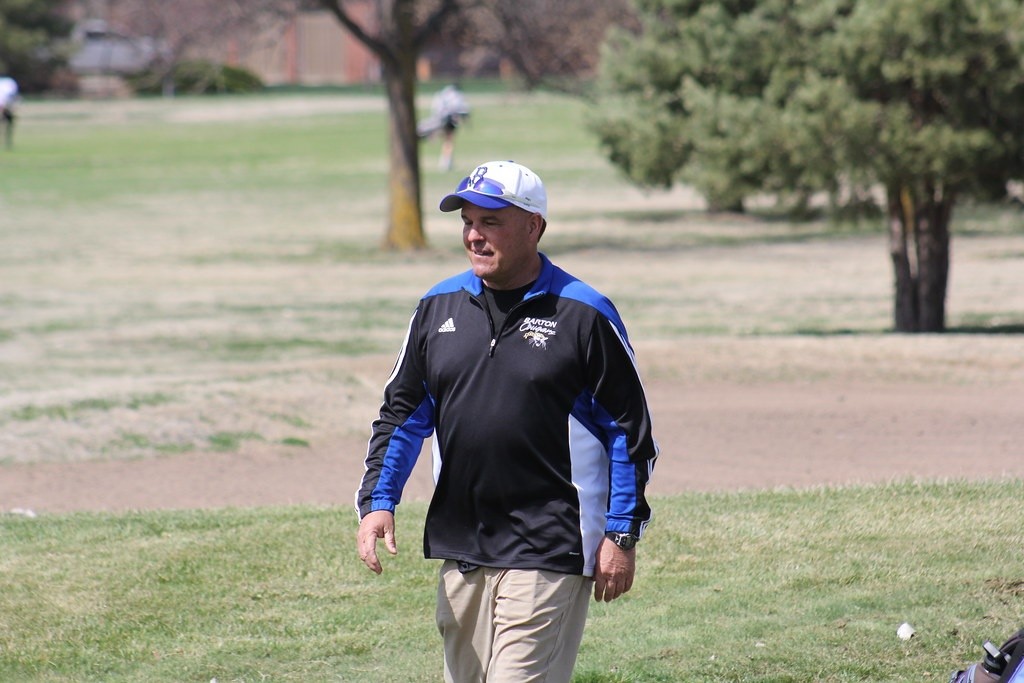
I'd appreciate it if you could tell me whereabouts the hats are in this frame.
[439,160,547,222]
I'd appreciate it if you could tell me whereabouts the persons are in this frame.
[952,625,1024,683]
[418,82,474,172]
[2,73,18,150]
[354,161,662,683]
